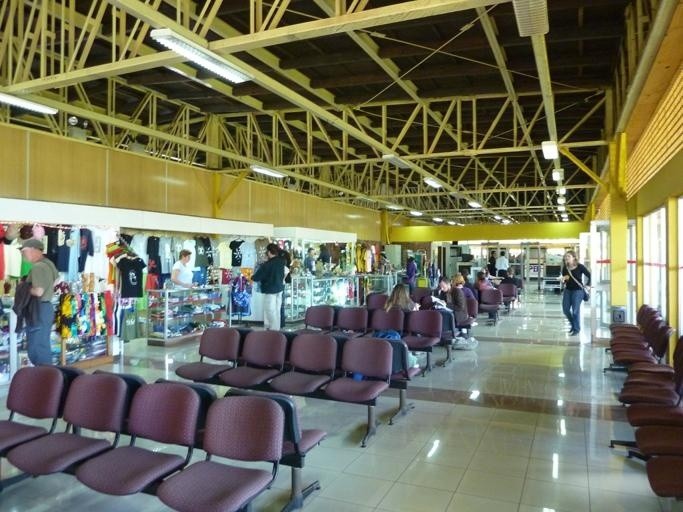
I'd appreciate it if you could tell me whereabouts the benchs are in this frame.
[1,366,326,510]
[365,282,519,351]
[176,303,453,448]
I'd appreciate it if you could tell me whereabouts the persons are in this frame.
[13,239,60,365]
[562,251,591,335]
[170,250,194,289]
[385,251,520,312]
[250,243,331,331]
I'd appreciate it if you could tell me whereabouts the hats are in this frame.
[17,240,44,254]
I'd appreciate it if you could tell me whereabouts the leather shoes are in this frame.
[569,327,579,337]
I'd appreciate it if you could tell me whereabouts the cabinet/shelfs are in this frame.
[282,270,410,322]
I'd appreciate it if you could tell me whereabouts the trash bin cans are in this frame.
[610,305,626,324]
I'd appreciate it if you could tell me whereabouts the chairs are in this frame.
[604,303,682,500]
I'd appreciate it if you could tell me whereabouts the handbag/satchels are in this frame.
[583,287,591,302]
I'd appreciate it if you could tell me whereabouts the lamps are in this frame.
[0,89,61,116]
[150,28,252,86]
[248,138,571,225]
